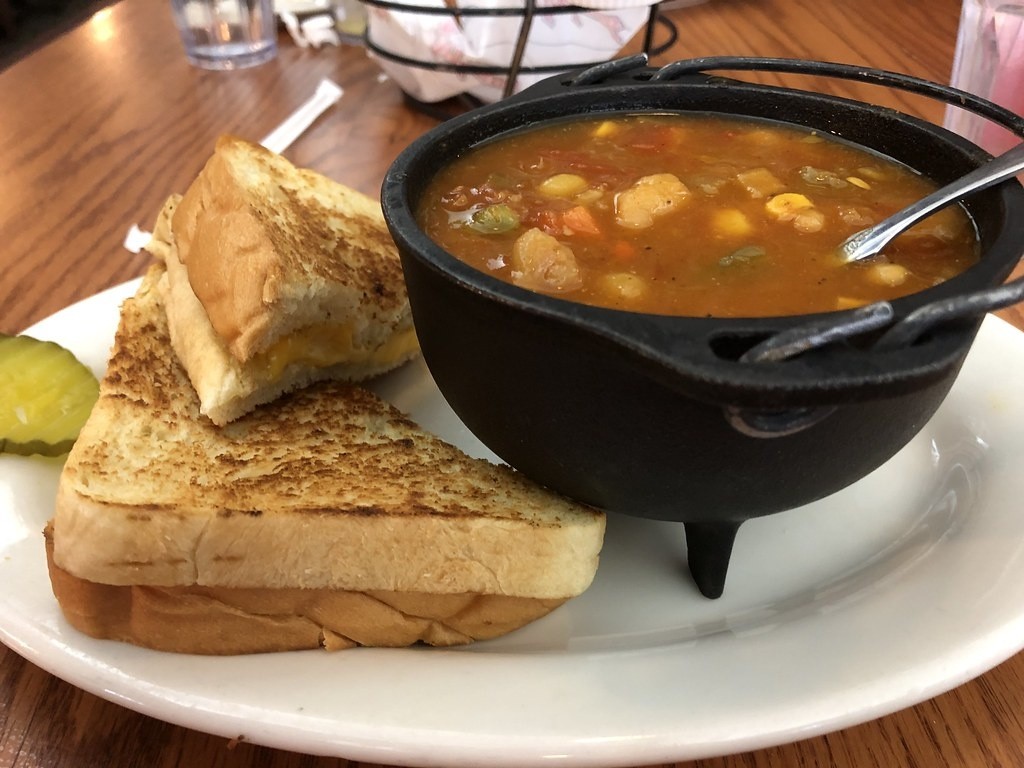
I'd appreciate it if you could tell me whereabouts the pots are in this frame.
[379,53,1024,600]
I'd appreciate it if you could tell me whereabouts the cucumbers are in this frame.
[0,332,100,457]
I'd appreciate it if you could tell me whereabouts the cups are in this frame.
[172,1,277,72]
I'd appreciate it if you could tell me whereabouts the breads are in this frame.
[44,134,606,649]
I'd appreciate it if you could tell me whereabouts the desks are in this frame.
[0,0,1024,768]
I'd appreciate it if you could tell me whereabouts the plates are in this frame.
[1,260,1023,768]
